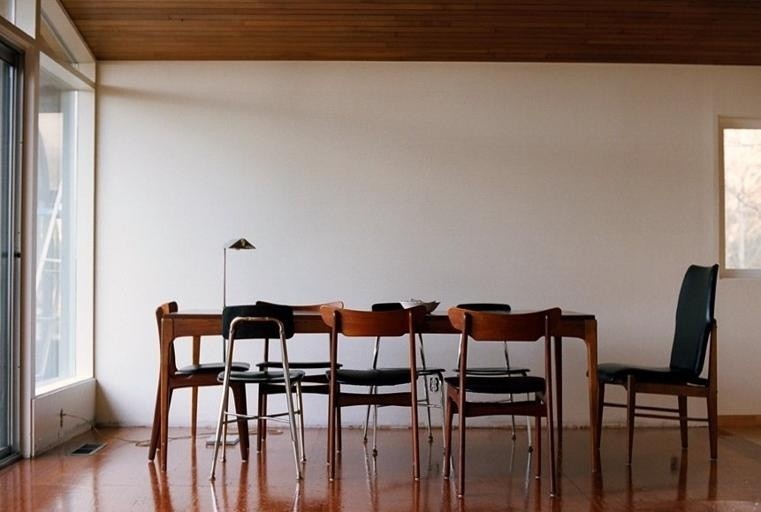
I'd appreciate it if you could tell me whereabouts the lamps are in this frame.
[205,238,255,445]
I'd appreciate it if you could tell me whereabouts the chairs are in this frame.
[208,305,301,480]
[362,301,445,453]
[255,301,343,453]
[451,302,532,453]
[585,264,720,466]
[148,301,249,461]
[318,304,426,482]
[442,307,561,499]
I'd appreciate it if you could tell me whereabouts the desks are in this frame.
[159,312,598,473]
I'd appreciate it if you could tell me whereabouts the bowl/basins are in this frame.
[399,300,440,315]
